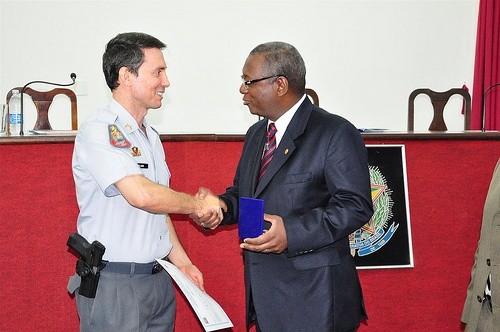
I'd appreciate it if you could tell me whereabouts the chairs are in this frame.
[407,88,471,132]
[5,86,78,131]
[258,88,319,120]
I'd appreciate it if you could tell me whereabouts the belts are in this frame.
[99,258,167,276]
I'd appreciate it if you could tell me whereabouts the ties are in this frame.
[256,123,278,191]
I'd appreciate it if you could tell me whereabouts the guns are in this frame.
[66,232,106,299]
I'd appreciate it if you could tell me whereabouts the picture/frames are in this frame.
[348,144,415,270]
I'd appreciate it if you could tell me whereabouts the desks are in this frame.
[0,129,500,332]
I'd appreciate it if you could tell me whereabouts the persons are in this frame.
[68,32,227,332]
[461,160,500,332]
[188,41,374,332]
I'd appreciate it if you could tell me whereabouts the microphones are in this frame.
[20,73,76,135]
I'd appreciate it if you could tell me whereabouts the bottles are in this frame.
[8,90,22,135]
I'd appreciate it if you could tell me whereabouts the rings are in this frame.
[200,223,205,227]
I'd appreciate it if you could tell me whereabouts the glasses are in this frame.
[241,74,287,89]
